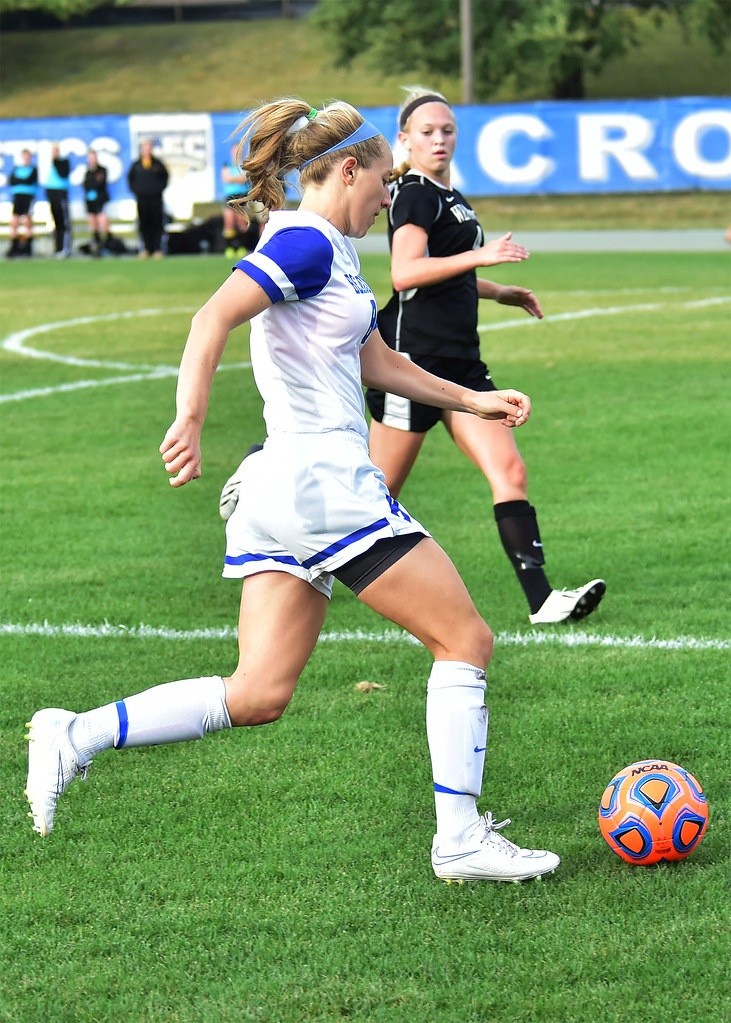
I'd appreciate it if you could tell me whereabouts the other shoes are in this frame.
[8,237,248,259]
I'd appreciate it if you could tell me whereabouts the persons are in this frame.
[83,150,112,254]
[42,146,71,260]
[128,139,169,259]
[222,142,283,258]
[25,100,563,885]
[246,84,608,624]
[7,149,38,259]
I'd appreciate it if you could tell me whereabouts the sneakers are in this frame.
[23,708,93,837]
[529,579,606,626]
[430,810,561,887]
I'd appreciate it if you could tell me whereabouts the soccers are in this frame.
[598,758,710,866]
